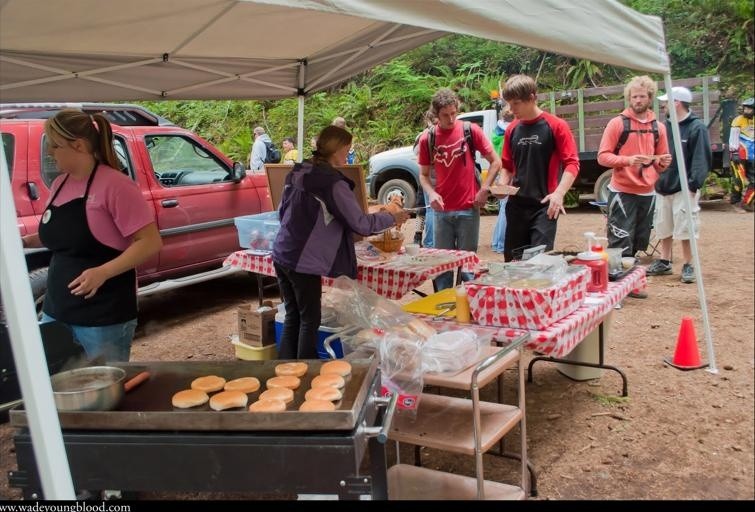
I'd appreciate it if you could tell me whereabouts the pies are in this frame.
[403,319,435,340]
[172,361,351,411]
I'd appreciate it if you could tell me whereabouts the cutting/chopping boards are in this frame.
[401,287,456,317]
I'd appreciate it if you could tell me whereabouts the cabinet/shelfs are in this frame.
[323,320,529,499]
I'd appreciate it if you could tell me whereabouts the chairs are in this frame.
[592,200,673,255]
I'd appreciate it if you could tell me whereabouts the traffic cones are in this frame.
[663,315,711,371]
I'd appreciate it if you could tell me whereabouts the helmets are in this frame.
[740,97,755,116]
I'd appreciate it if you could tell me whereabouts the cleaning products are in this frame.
[576,232,608,291]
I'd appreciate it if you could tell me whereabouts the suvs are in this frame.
[1,106,275,348]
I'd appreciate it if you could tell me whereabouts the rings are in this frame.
[556,206,561,210]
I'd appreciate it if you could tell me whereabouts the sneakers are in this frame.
[645,261,697,284]
[628,290,648,298]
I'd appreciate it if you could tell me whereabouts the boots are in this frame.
[413,231,424,248]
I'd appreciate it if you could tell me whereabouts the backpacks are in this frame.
[256,138,282,164]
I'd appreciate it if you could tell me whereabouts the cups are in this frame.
[405,244,422,255]
[609,247,623,274]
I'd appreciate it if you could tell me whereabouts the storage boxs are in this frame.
[234,212,280,250]
[275,310,357,364]
[230,339,276,361]
[238,304,277,347]
[461,268,589,329]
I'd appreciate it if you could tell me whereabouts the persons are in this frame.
[248,126,282,173]
[416,86,502,293]
[332,116,356,166]
[279,135,299,164]
[411,107,441,248]
[644,86,713,284]
[308,134,319,153]
[498,73,581,263]
[595,75,673,308]
[490,102,518,255]
[728,97,755,212]
[19,108,165,377]
[269,125,413,360]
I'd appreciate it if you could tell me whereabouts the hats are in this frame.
[656,86,693,104]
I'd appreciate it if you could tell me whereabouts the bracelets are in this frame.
[19,236,27,249]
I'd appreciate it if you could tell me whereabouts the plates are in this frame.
[488,186,520,196]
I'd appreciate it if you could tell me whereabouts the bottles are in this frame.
[455,279,470,324]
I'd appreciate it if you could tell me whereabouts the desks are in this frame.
[411,267,652,494]
[223,243,478,306]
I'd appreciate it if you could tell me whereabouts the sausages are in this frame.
[125,372,149,390]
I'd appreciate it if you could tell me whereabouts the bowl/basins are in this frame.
[48,366,125,412]
[622,257,636,268]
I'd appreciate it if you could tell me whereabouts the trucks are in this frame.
[365,75,737,219]
